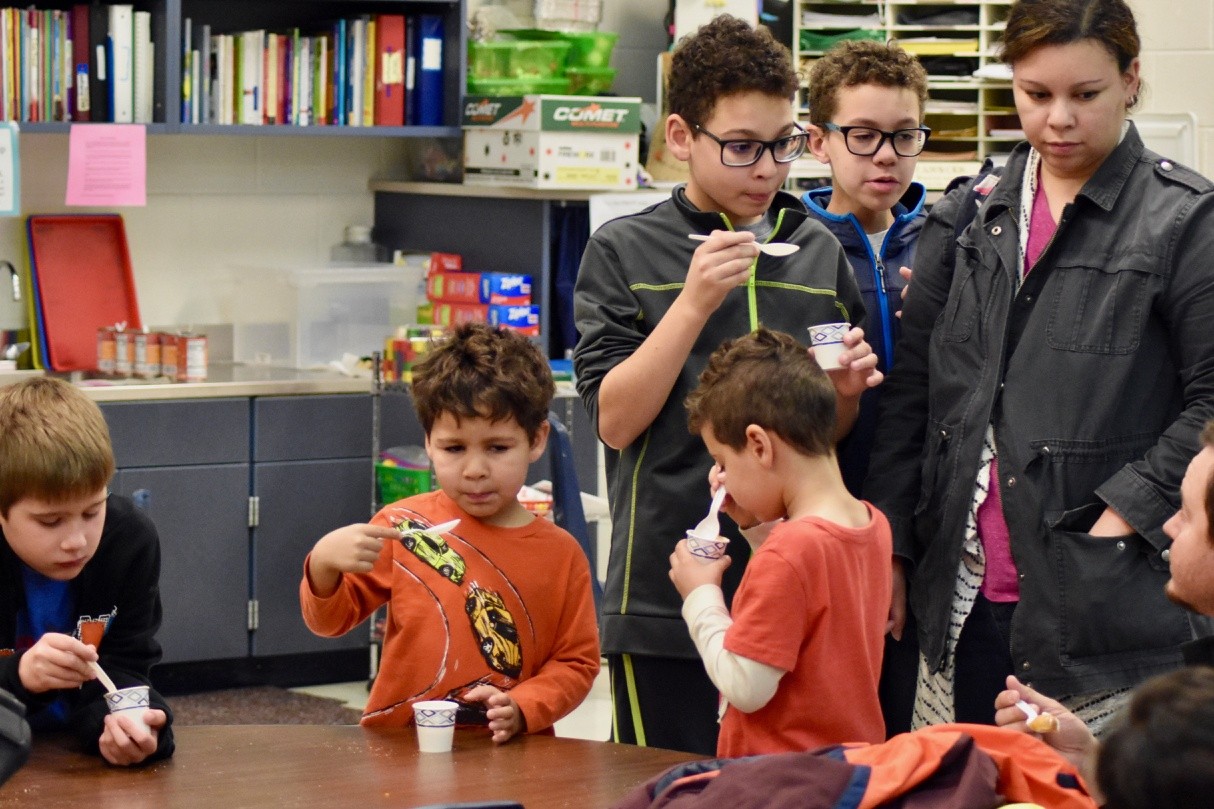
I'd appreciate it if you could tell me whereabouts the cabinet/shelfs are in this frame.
[54,389,613,695]
[0,0,464,139]
[791,1,1076,190]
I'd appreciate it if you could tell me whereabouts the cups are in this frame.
[687,530,729,561]
[410,700,458,755]
[805,321,851,371]
[104,685,150,737]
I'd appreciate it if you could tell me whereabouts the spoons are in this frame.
[400,519,460,536]
[1017,699,1060,734]
[687,233,800,257]
[696,482,730,539]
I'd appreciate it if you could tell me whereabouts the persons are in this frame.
[993,414,1214,809]
[863,1,1213,740]
[299,322,601,746]
[0,377,175,769]
[573,13,933,758]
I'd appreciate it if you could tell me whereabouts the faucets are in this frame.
[0,259,31,360]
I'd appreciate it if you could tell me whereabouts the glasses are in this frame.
[825,120,931,157]
[682,119,811,167]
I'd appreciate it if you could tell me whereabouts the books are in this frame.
[0,0,445,127]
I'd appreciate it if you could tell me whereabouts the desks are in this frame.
[0,726,724,809]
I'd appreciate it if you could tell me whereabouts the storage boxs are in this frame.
[462,27,641,189]
[94,247,541,383]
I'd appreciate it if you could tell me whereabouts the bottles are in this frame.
[328,227,378,263]
